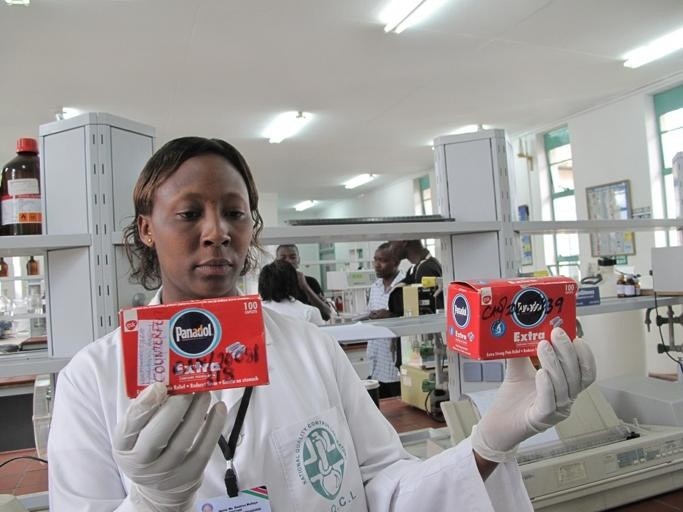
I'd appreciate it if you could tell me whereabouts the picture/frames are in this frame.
[519,204,533,266]
[585,179,636,257]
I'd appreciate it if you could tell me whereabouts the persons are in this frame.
[366,243,408,400]
[370,240,443,369]
[47,138,597,512]
[276,244,332,320]
[202,503,214,512]
[257,261,325,326]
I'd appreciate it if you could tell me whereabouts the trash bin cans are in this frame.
[362,379,380,408]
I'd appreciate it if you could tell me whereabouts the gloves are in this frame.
[109,383,229,511]
[472,327,598,466]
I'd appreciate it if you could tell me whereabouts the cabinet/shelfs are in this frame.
[0,113,683,400]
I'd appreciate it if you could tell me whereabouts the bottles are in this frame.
[26,256,38,275]
[0,137,43,236]
[616,275,641,298]
[0,257,8,277]
